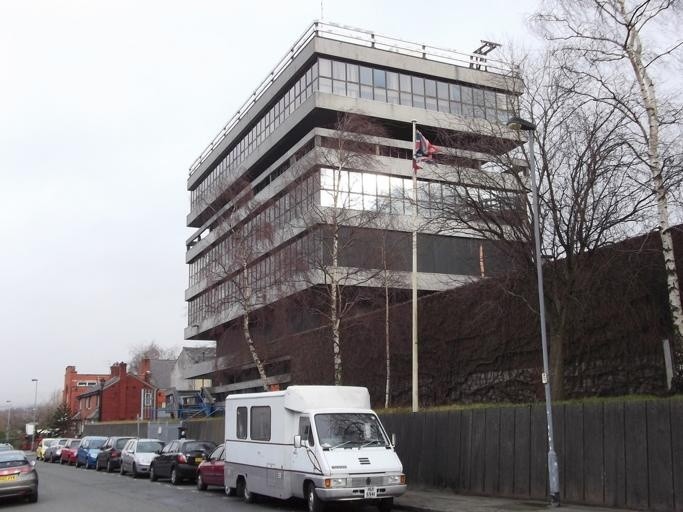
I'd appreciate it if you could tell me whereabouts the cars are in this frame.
[36,437,223,491]
[0,450,39,503]
[0,442,14,452]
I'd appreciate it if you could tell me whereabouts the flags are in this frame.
[410,125,441,173]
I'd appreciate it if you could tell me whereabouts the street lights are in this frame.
[31,379,39,452]
[506,115,561,508]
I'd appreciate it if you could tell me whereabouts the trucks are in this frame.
[224,386,407,512]
